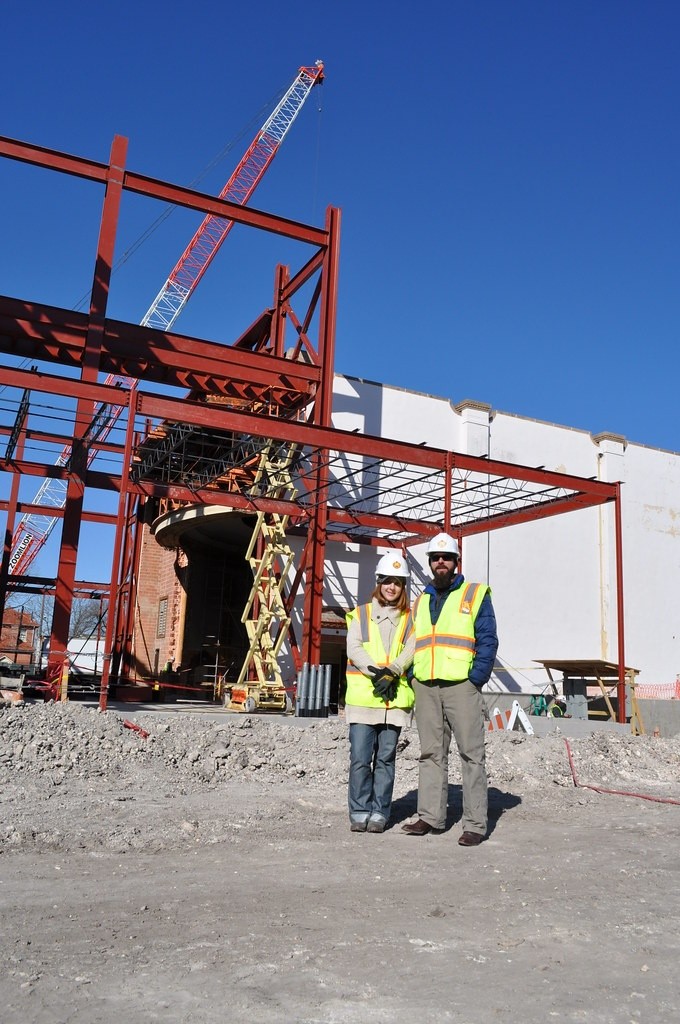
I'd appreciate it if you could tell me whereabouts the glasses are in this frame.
[430,554,454,562]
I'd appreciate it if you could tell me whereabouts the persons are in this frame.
[344,553,415,832]
[547,696,572,718]
[402,533,499,847]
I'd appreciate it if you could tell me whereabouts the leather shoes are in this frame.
[458,831,484,845]
[402,819,442,835]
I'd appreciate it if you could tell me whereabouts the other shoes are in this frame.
[367,813,389,833]
[350,813,370,831]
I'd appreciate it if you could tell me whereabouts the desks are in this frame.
[532,658,641,722]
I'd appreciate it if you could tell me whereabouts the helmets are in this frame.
[375,553,411,577]
[425,533,460,557]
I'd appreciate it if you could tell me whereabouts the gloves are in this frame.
[368,665,396,697]
[381,684,394,701]
